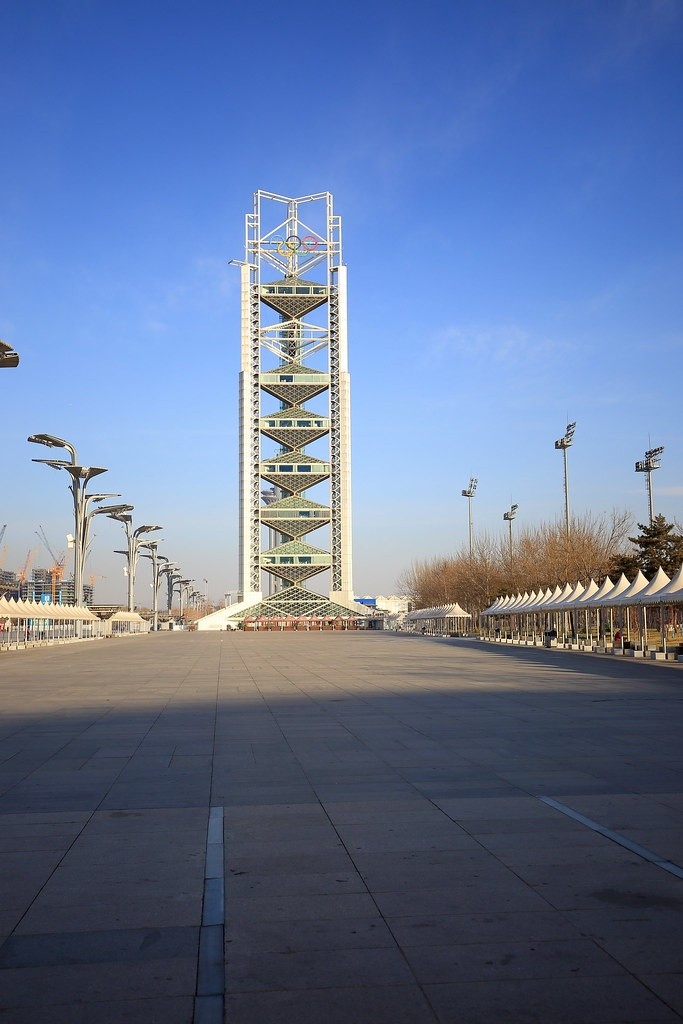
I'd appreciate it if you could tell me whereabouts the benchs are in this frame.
[477,630,683,663]
[0,634,97,651]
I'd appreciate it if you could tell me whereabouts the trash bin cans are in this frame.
[494,627,501,641]
[544,629,557,648]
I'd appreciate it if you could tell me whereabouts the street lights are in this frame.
[462,478,478,565]
[27,435,134,638]
[556,420,577,546]
[107,515,207,632]
[503,504,518,577]
[636,446,664,532]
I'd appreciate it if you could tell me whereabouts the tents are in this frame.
[405,602,471,635]
[480,563,683,655]
[244,614,358,630]
[0,596,101,646]
[106,612,149,635]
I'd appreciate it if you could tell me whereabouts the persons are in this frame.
[615,629,625,642]
[24,626,30,641]
[395,623,398,632]
[495,627,499,637]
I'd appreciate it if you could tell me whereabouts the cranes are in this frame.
[17,524,97,605]
